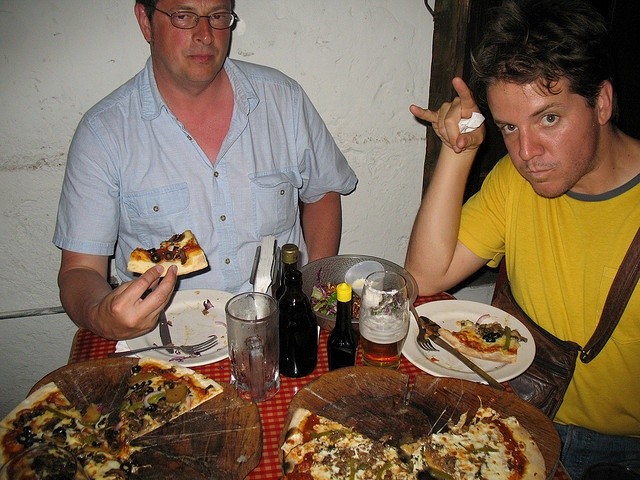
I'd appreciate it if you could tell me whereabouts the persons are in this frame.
[51,0,360,341]
[398,1,640,480]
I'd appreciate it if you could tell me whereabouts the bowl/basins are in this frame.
[345,259,385,299]
[297,254,418,337]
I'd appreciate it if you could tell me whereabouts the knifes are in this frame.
[158,310,174,354]
[422,328,506,393]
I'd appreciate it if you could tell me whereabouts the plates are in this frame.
[397,299,537,386]
[125,289,251,368]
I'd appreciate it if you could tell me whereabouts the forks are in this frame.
[106,337,219,359]
[408,296,440,352]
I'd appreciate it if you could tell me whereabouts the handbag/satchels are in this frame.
[490,258,583,421]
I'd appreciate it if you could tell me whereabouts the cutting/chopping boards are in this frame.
[26,356,263,480]
[277,365,562,480]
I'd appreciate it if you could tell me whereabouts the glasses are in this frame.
[153,9,237,30]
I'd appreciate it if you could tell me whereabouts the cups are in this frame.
[226,291,281,402]
[359,271,411,371]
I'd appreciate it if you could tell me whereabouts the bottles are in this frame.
[278,269,316,378]
[276,243,299,309]
[327,281,358,371]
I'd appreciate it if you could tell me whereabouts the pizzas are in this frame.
[437,322,528,364]
[126,230,209,277]
[0,357,225,479]
[282,405,547,480]
